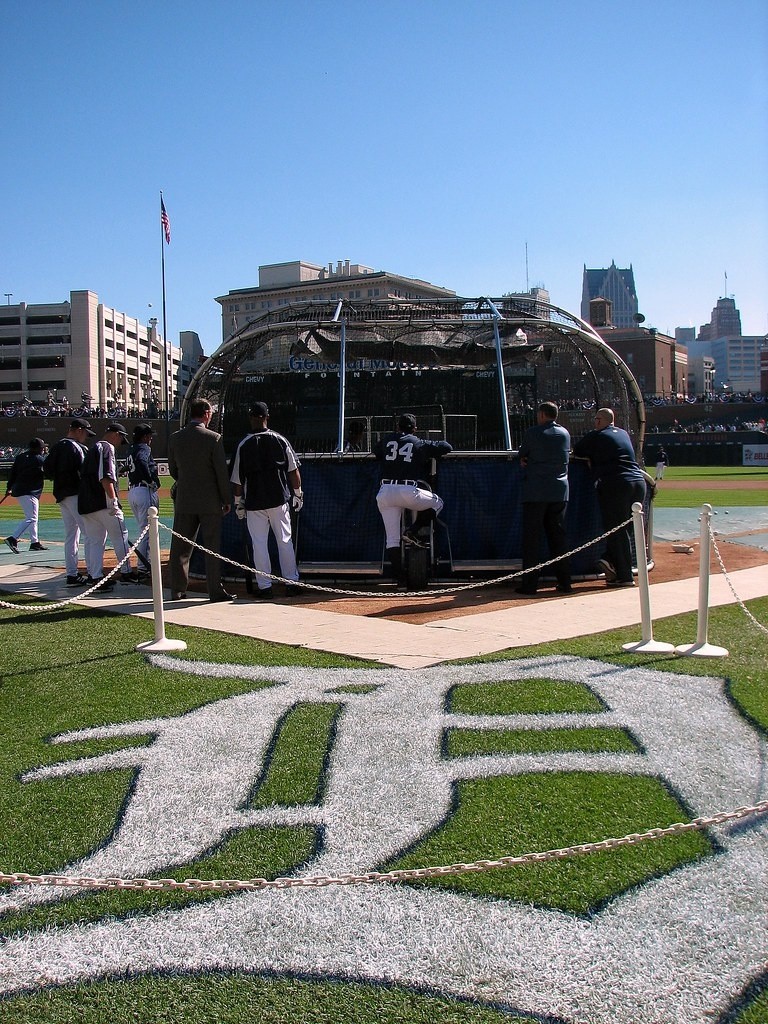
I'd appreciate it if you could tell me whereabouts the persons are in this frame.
[124,425,161,577]
[334,421,367,451]
[230,402,307,599]
[513,400,574,595]
[78,423,150,593]
[0,446,49,458]
[643,389,768,404]
[53,418,106,586]
[654,420,705,433]
[573,408,646,586]
[3,437,49,554]
[741,420,768,435]
[166,398,237,602]
[654,447,669,480]
[704,422,740,432]
[508,392,638,415]
[371,414,454,593]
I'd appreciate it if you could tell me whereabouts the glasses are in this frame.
[594,417,602,420]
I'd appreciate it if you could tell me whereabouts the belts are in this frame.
[130,484,150,487]
[381,481,417,486]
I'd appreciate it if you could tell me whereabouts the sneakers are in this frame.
[65,568,152,593]
[392,579,407,591]
[29,542,50,551]
[4,536,20,554]
[401,529,430,549]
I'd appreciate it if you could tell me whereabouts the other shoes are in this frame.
[285,586,304,597]
[252,586,273,599]
[606,578,636,585]
[514,586,539,595]
[556,583,573,593]
[172,590,187,600]
[660,477,662,480]
[210,587,238,602]
[655,478,657,480]
[597,558,617,580]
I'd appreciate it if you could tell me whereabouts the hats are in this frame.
[70,419,97,437]
[132,423,155,435]
[30,438,49,449]
[105,423,130,444]
[248,402,268,419]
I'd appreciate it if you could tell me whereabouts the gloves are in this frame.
[108,498,118,516]
[292,487,304,512]
[233,496,245,520]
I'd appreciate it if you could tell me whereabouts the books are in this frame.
[0,400,181,419]
